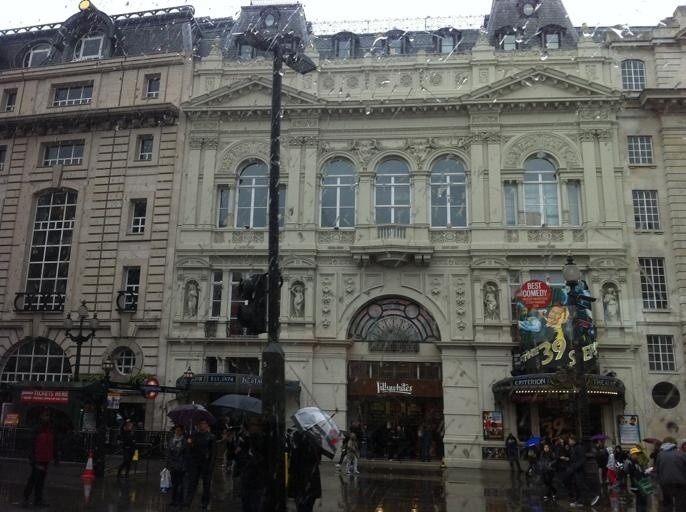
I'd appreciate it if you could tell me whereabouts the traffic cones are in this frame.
[81,452,97,479]
[82,478,94,506]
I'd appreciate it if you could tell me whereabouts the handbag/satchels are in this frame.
[637,479,654,498]
[158,467,172,495]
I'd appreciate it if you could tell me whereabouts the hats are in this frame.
[629,447,641,454]
[661,441,676,452]
[676,438,686,451]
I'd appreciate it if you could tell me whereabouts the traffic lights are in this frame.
[237,272,267,337]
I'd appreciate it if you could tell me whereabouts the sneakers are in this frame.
[18,496,50,508]
[168,501,208,512]
[589,495,599,506]
[570,500,585,508]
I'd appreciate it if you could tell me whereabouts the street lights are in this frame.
[234,30,317,511]
[560,256,596,444]
[64,300,99,382]
[102,355,114,416]
[184,366,195,438]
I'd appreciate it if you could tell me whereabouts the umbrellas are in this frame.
[210,389,262,431]
[290,405,341,460]
[166,400,221,428]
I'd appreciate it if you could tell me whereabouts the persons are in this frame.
[188,281,197,318]
[290,283,304,319]
[483,413,502,437]
[15,415,362,512]
[482,284,498,320]
[602,285,620,323]
[363,410,444,464]
[503,431,686,512]
[628,416,637,426]
